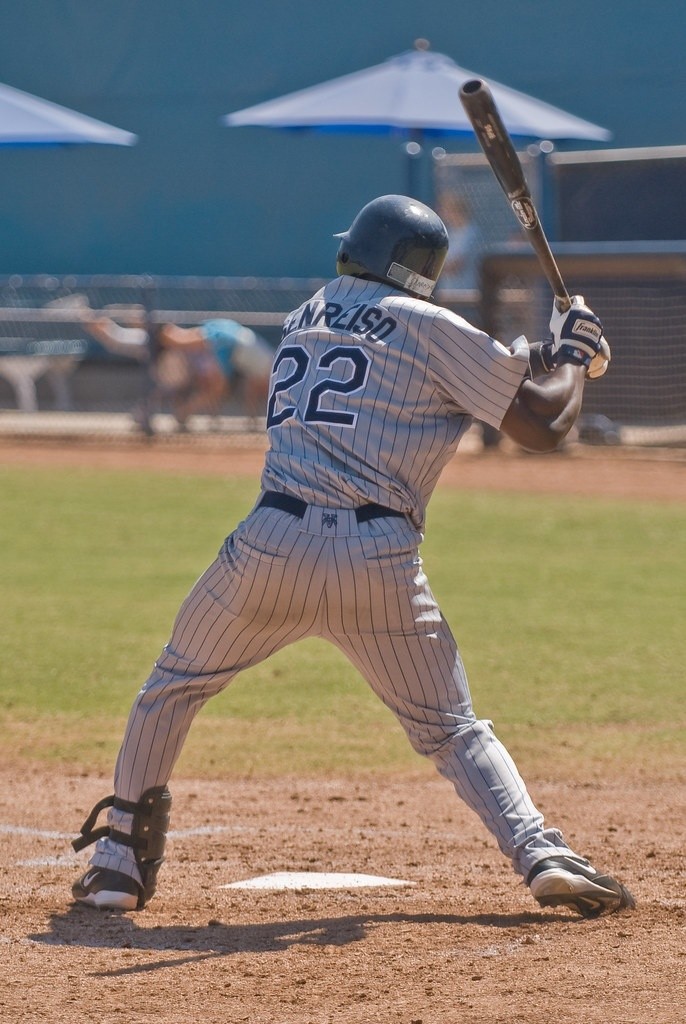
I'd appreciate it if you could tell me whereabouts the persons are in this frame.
[71,194,636,918]
[77,301,278,435]
[434,183,488,455]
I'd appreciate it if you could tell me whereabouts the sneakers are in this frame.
[72,867,139,911]
[528,855,635,917]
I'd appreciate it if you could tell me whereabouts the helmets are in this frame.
[334,193,450,299]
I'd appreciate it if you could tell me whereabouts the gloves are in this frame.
[543,293,610,381]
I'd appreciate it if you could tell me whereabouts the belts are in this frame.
[258,489,404,525]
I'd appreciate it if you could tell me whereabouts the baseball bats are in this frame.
[457,77,572,316]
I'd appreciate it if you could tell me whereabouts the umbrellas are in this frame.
[0,82,139,148]
[221,38,614,211]
